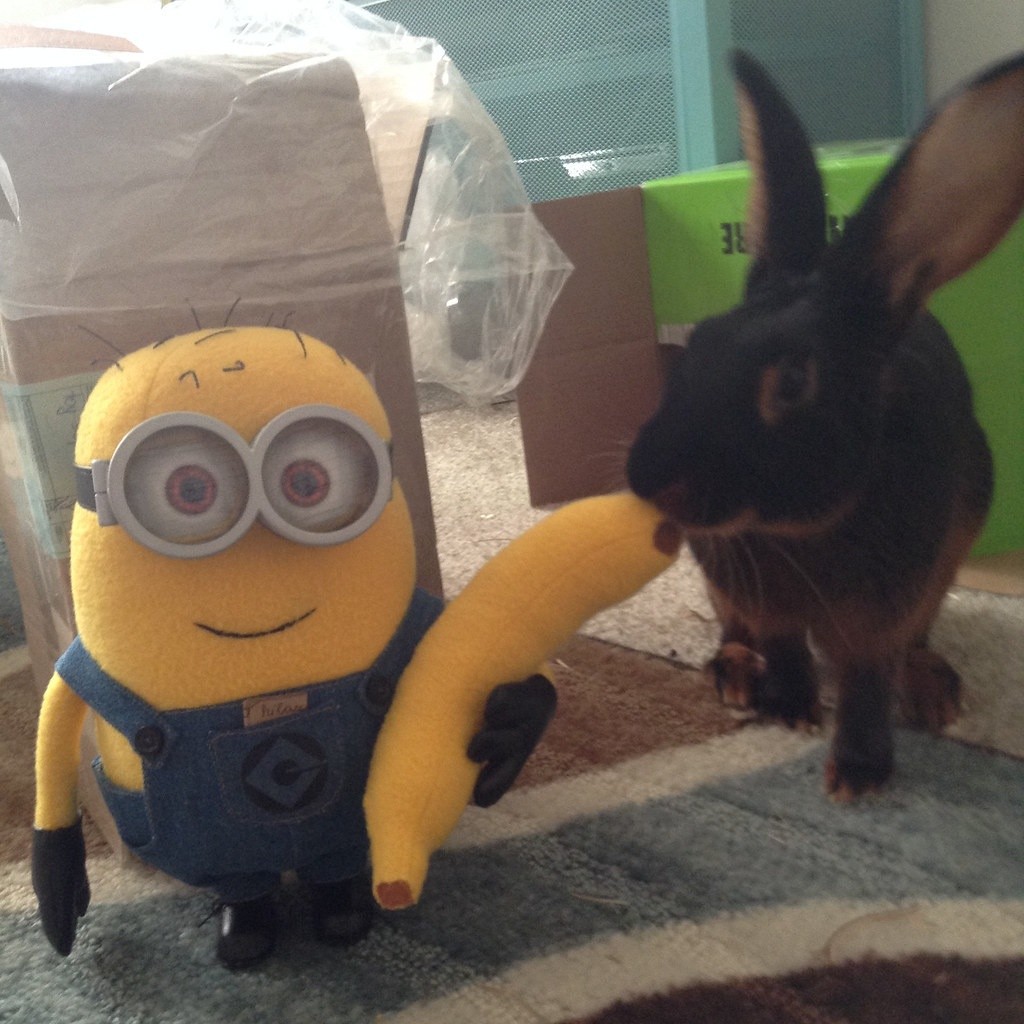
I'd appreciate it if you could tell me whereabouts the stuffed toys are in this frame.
[23,311,565,973]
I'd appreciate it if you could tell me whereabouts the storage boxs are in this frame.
[0,34,444,866]
[503,141,1023,599]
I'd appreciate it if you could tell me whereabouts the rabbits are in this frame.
[627,50,1024,811]
